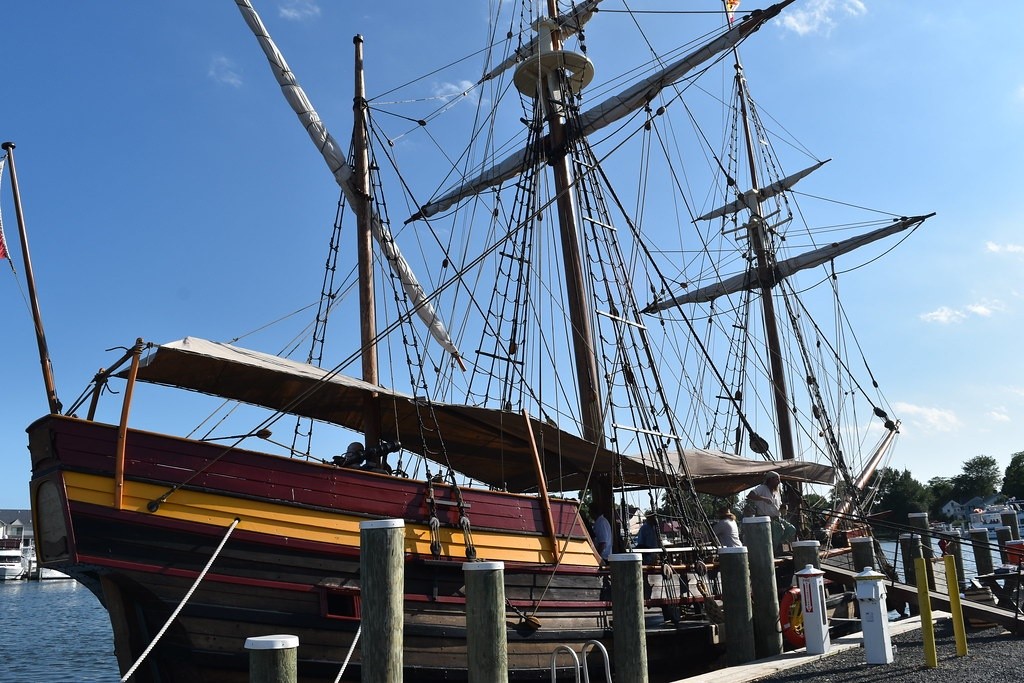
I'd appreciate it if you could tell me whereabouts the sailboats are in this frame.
[0,1,943,682]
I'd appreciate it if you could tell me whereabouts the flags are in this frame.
[725,0,740,25]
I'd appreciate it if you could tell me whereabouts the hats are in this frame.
[721,508,734,516]
[644,510,654,517]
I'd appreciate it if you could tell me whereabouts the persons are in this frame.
[588,502,612,566]
[638,510,658,566]
[747,471,796,558]
[712,507,743,548]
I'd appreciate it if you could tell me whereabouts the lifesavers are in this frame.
[780,587,805,647]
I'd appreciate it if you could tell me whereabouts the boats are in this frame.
[0,549,25,580]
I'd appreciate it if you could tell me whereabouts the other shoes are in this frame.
[781,551,793,556]
